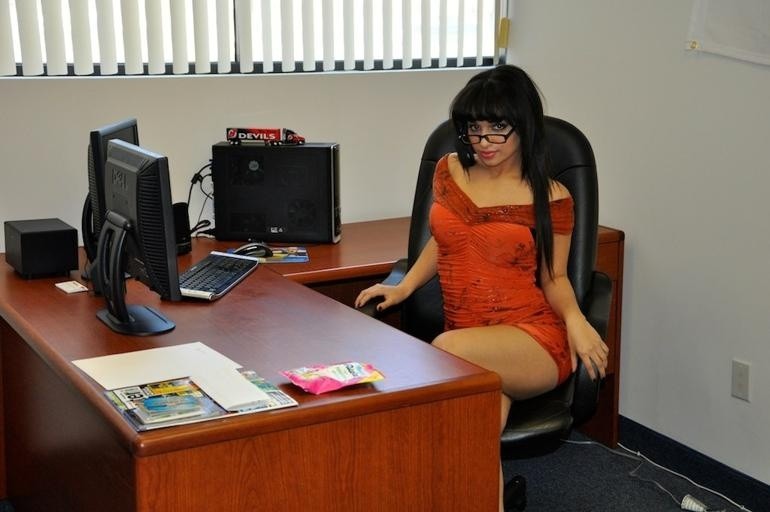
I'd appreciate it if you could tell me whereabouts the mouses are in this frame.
[233,242,273,257]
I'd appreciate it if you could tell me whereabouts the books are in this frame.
[104,367,300,435]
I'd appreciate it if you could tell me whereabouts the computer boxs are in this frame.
[212,141,342,243]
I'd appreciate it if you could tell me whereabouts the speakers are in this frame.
[172,202,192,256]
[3,218,79,280]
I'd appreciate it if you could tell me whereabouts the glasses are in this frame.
[459,123,517,145]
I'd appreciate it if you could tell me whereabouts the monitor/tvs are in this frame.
[80,119,139,281]
[96,139,179,337]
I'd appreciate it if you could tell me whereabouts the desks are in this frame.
[1,216,627,512]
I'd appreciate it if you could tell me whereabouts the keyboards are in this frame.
[178,251,258,300]
